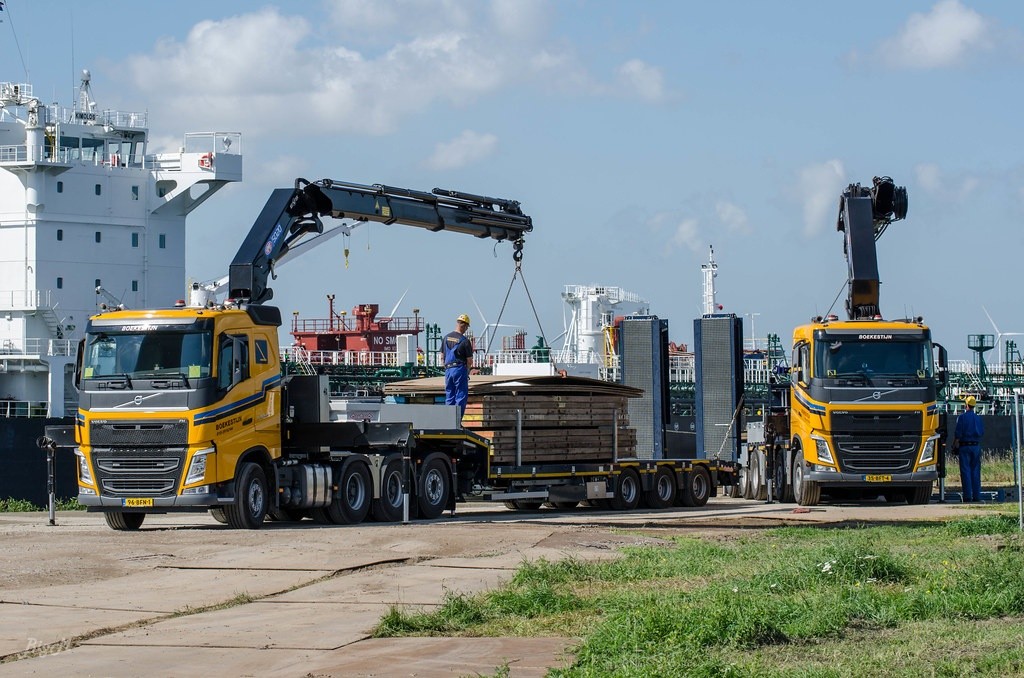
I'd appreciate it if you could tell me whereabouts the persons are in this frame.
[951,396,985,503]
[439,315,474,425]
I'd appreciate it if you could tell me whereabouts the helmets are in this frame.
[965,396,977,407]
[457,314,470,327]
[416,348,422,353]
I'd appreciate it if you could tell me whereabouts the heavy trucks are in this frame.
[72,178,743,530]
[723,176,950,505]
